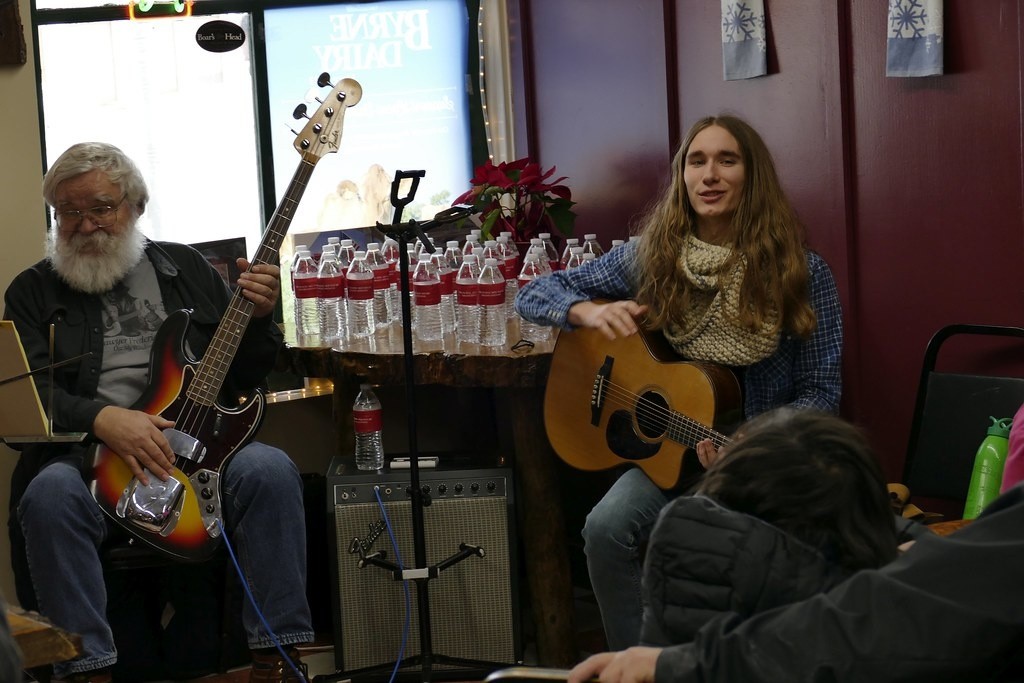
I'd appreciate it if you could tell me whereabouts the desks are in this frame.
[277,323,581,670]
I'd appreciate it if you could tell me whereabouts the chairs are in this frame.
[901,322,1024,501]
[101,534,236,674]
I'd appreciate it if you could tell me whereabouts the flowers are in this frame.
[451,156,579,241]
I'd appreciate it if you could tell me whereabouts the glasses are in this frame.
[53,194,128,231]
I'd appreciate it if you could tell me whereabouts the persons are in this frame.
[0,143,316,683]
[569,407,1024,683]
[514,116,842,654]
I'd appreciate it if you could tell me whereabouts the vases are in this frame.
[515,237,563,262]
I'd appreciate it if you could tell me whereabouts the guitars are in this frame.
[88,70,364,562]
[542,298,736,491]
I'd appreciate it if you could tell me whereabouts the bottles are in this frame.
[291,230,639,347]
[353,384,384,470]
[963,416,1013,519]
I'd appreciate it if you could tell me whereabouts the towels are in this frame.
[885,1,945,77]
[720,0,767,80]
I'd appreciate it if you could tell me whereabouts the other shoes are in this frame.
[249,647,307,682]
